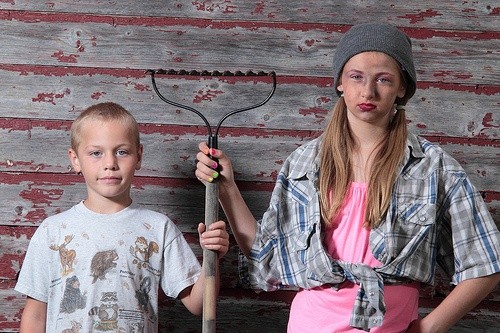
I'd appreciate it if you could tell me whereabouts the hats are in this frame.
[333,23,416,102]
